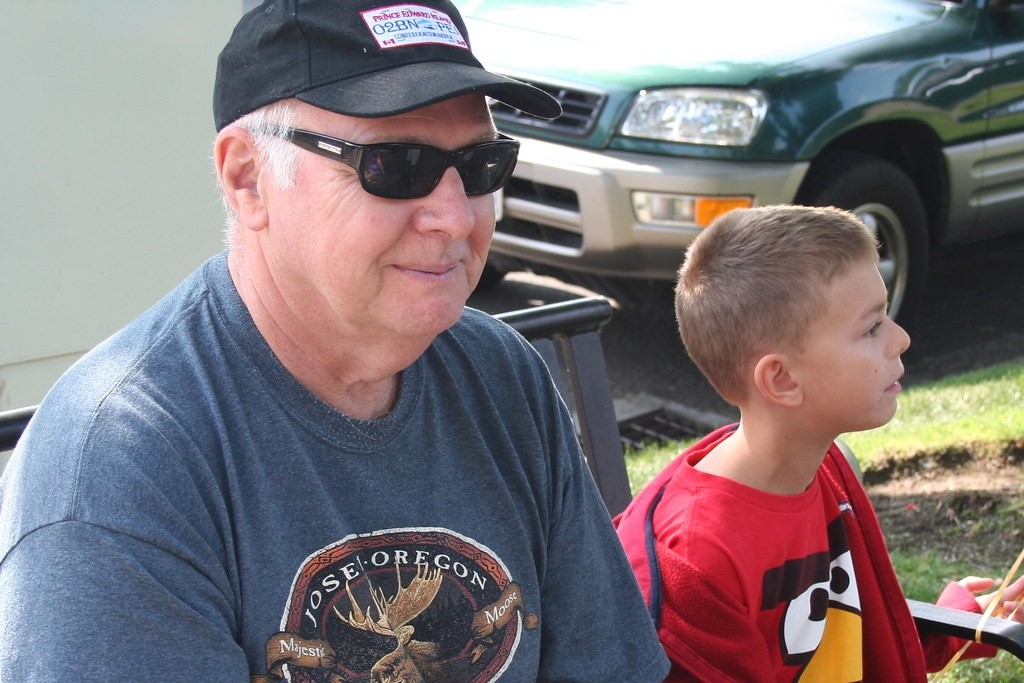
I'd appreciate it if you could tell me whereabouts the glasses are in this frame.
[256,122,520,200]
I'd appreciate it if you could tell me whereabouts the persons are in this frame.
[0,0,673,683]
[609,204,1024,683]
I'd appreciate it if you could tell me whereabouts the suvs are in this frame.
[450,0,1024,335]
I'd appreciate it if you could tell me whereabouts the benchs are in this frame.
[0,297,1024,667]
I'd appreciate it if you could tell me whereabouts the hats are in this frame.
[214,0,563,132]
